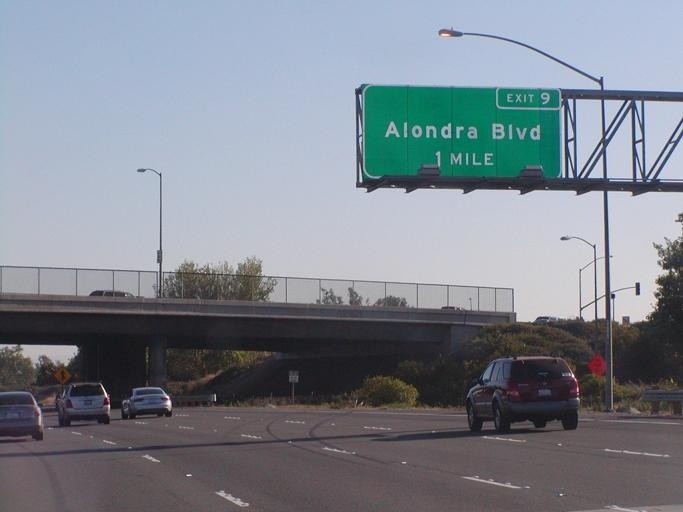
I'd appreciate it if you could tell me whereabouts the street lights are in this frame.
[559,235,599,360]
[577,254,612,318]
[135,167,164,299]
[437,26,617,409]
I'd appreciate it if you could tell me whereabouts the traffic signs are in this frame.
[360,82,561,179]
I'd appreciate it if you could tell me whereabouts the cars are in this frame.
[89,289,143,298]
[532,316,553,324]
[441,306,464,310]
[121,385,172,418]
[0,391,44,440]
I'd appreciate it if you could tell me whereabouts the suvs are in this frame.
[55,382,111,424]
[463,353,579,430]
